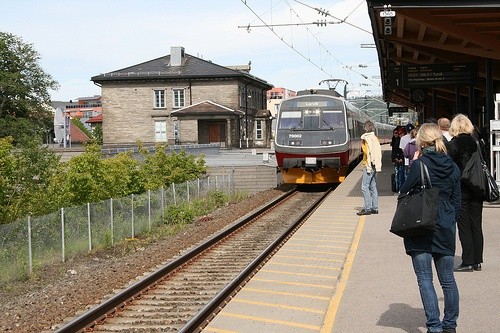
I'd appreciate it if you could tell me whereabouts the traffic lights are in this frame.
[380,6,396,36]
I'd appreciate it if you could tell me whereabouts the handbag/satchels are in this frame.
[449,138,486,198]
[390,159,440,238]
[482,160,500,202]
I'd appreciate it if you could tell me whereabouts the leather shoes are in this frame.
[452,265,473,271]
[473,263,482,271]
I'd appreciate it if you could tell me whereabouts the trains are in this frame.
[273,77,399,192]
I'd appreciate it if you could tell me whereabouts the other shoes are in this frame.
[356,209,371,216]
[371,210,379,214]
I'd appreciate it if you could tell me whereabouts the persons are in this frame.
[404,128,419,168]
[390,123,462,333]
[391,123,415,193]
[438,118,453,140]
[357,121,382,215]
[447,113,499,271]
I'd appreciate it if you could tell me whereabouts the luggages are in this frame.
[391,166,400,193]
